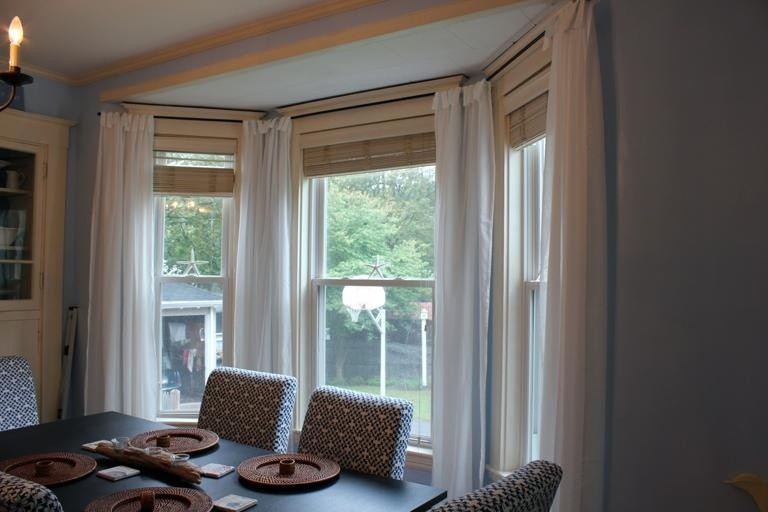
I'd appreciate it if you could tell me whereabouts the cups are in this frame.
[35,460,54,477]
[144,447,162,457]
[111,436,130,449]
[140,491,155,512]
[170,454,189,465]
[156,435,171,448]
[279,459,295,475]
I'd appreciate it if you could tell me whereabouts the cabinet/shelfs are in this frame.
[0,108,76,424]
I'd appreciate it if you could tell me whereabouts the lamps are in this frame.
[0,15,33,112]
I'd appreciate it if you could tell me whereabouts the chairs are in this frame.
[0,355,40,431]
[197,366,297,454]
[429,460,563,512]
[0,471,63,512]
[297,385,414,481]
